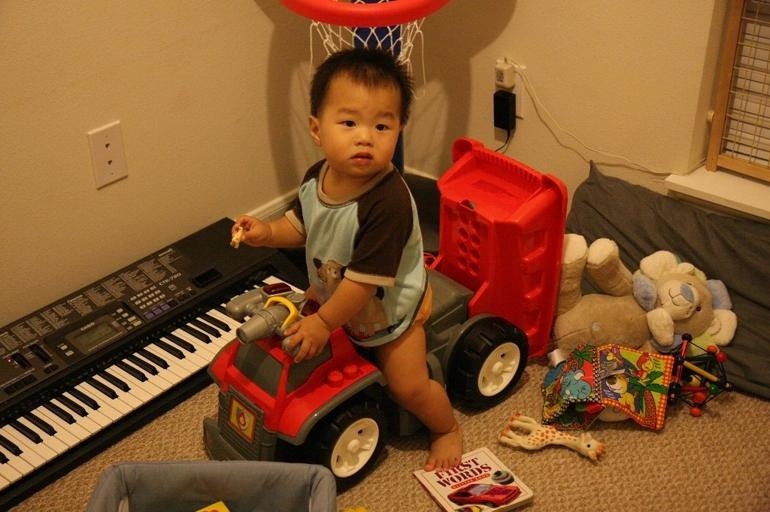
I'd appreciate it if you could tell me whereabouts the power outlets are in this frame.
[86,119,128,191]
[498,64,526,120]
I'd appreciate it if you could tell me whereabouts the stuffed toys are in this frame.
[548,234,737,369]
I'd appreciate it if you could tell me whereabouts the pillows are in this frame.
[565,159,770,401]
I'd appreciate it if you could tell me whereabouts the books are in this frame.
[412,446,534,512]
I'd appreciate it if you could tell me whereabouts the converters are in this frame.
[495,63,516,90]
[491,89,517,134]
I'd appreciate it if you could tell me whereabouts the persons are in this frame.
[231,48,464,472]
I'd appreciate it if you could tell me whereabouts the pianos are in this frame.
[0,216,315,510]
